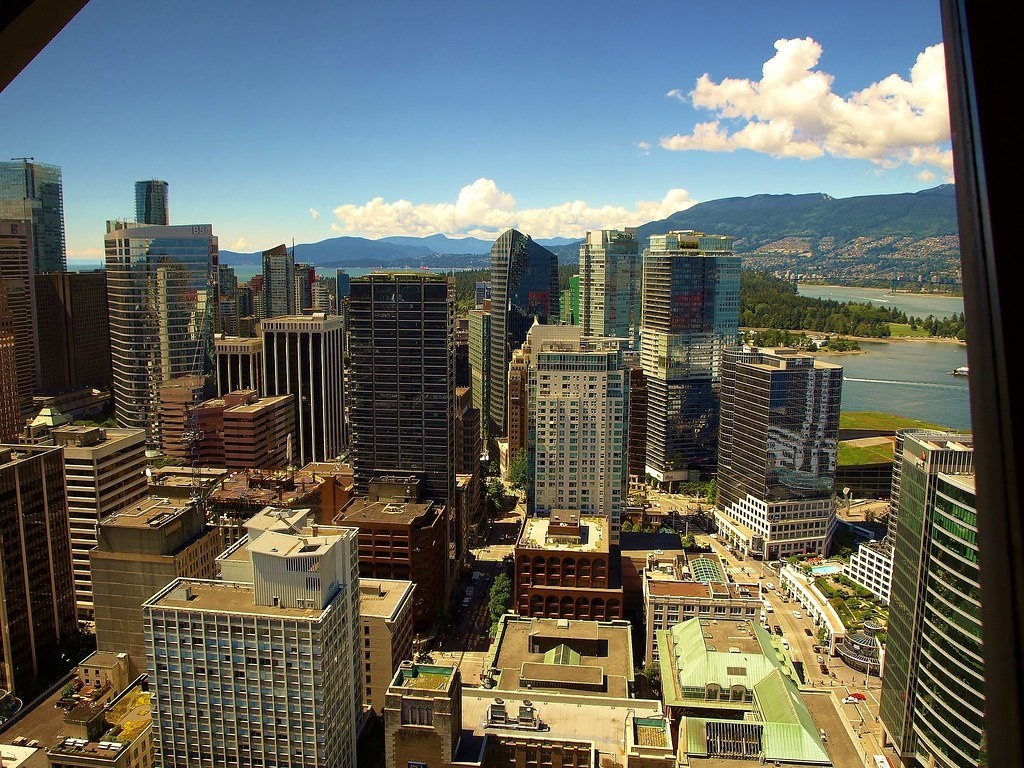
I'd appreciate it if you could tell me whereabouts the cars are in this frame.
[762,623,771,631]
[812,644,821,653]
[847,693,867,700]
[765,582,776,590]
[780,595,789,603]
[780,637,789,650]
[758,610,767,621]
[774,625,784,637]
[499,533,505,540]
[820,664,830,674]
[764,600,774,613]
[842,696,860,704]
[667,515,708,535]
[793,610,802,619]
[804,628,813,636]
[817,654,824,664]
[709,531,744,561]
